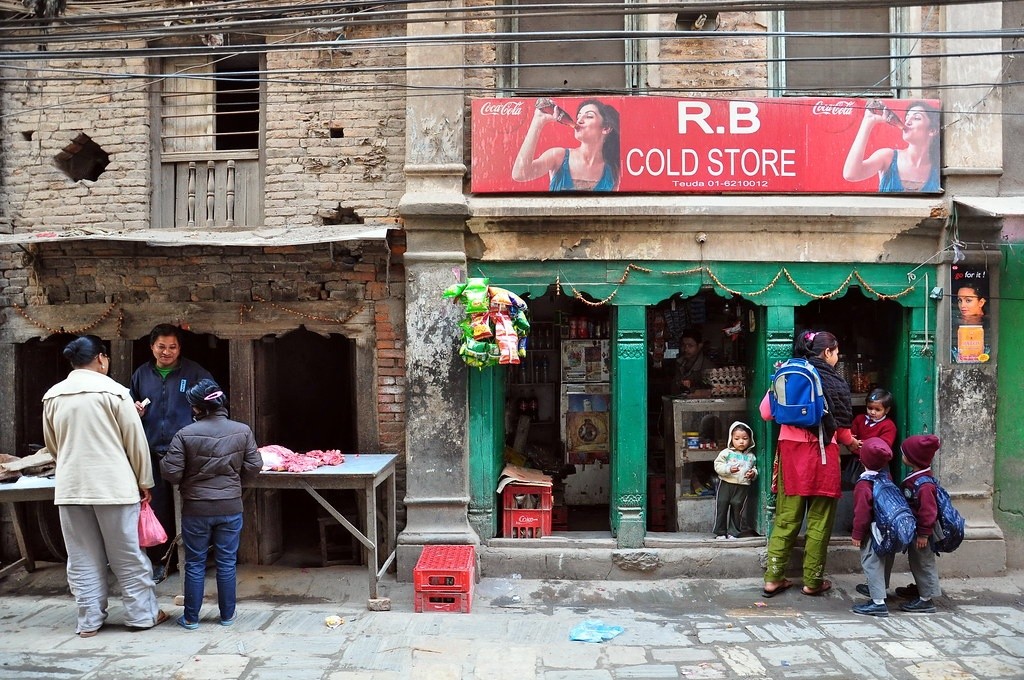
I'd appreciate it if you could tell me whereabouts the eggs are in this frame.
[716,386,740,392]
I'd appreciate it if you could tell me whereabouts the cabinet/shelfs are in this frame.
[664,395,745,533]
[506,323,556,424]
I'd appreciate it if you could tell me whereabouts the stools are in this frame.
[318,509,359,565]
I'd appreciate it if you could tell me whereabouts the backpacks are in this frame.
[913,476,965,558]
[769,355,826,427]
[855,474,917,555]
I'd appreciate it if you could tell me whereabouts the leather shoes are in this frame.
[856,584,871,597]
[895,584,920,598]
[900,597,936,613]
[852,599,888,616]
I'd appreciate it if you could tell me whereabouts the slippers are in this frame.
[763,579,793,597]
[220,611,237,625]
[79,630,98,637]
[134,609,170,629]
[801,580,831,595]
[177,615,198,629]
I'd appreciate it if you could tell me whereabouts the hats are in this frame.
[901,435,940,468]
[860,437,892,471]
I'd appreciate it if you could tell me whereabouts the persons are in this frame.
[952,282,991,325]
[712,421,758,540]
[42,335,170,638]
[843,388,897,491]
[159,379,263,630]
[895,435,942,613]
[130,323,213,580]
[842,101,940,192]
[852,437,895,617]
[511,98,621,191]
[759,331,861,598]
[672,329,714,396]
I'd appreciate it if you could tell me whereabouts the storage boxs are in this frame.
[504,485,552,540]
[414,547,474,614]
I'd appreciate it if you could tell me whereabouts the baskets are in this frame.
[413,544,474,613]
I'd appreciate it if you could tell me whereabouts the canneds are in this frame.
[958,325,984,360]
[569,314,611,338]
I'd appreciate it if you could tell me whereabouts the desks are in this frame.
[0,454,396,598]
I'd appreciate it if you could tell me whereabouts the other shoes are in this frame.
[714,534,726,540]
[728,535,737,538]
[153,564,170,583]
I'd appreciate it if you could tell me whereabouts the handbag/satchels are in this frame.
[138,502,167,548]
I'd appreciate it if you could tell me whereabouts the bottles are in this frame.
[568,316,610,339]
[529,328,552,349]
[507,355,549,384]
[834,352,871,393]
[535,98,579,129]
[517,389,540,423]
[864,98,908,131]
